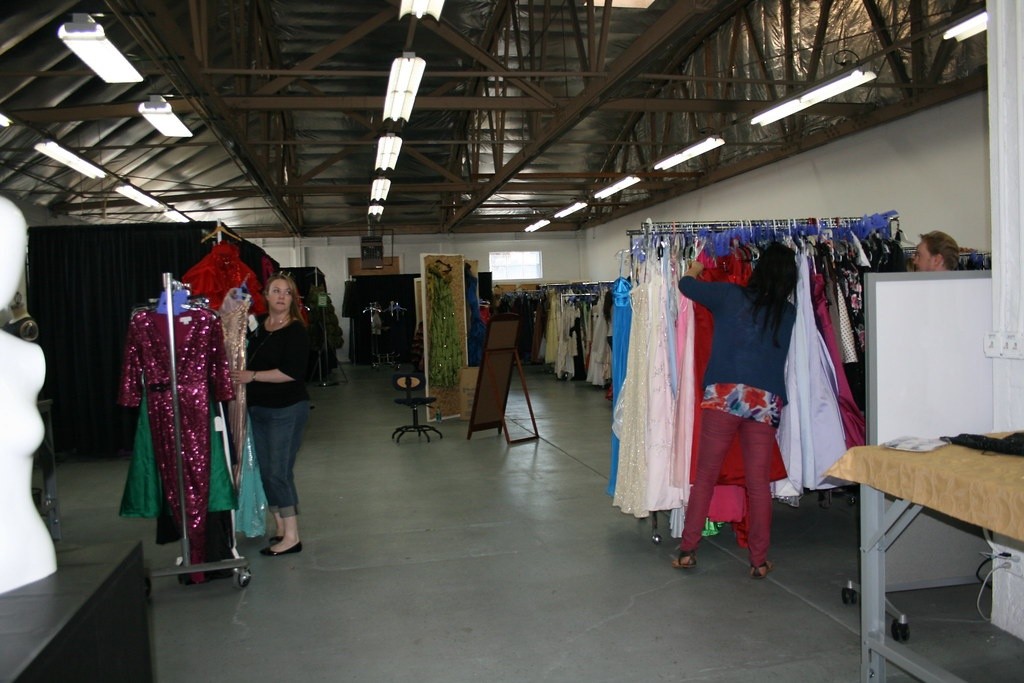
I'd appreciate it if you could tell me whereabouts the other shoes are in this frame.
[750,561,773,579]
[270,535,285,543]
[258,542,302,555]
[672,554,696,569]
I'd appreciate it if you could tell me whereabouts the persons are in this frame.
[914,230,959,272]
[0,195,57,594]
[603,290,613,401]
[494,298,509,314]
[673,242,799,579]
[3,302,38,345]
[464,263,487,367]
[409,322,424,371]
[228,274,310,556]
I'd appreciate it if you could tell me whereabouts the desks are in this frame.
[827,433,1024,683]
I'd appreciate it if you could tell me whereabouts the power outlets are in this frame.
[1005,552,1023,577]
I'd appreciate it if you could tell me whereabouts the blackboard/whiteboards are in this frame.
[863,271,994,593]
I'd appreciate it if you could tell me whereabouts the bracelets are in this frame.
[252,371,257,381]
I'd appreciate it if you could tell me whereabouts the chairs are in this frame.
[391,372,443,444]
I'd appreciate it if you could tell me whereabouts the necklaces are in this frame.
[270,314,289,336]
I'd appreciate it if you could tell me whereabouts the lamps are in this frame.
[164,209,190,223]
[366,132,402,217]
[748,65,878,127]
[398,0,445,22]
[138,93,195,138]
[942,11,989,42]
[33,137,107,179]
[112,181,159,208]
[526,173,643,232]
[382,51,426,123]
[57,14,145,84]
[653,134,726,171]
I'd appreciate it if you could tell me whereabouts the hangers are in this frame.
[363,302,407,313]
[152,224,243,313]
[611,217,890,265]
